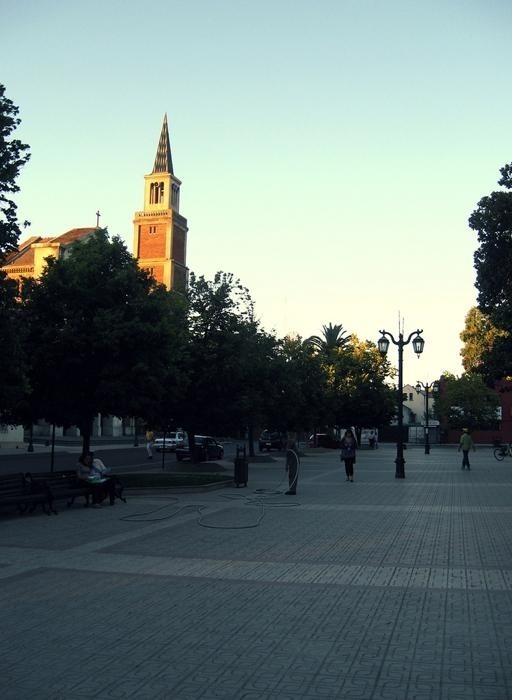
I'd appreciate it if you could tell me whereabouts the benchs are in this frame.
[0,470,124,518]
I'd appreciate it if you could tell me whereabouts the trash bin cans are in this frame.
[234,456,249,488]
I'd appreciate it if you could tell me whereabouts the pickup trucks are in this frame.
[175,435,225,461]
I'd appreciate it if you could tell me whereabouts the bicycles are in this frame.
[493,440,512,462]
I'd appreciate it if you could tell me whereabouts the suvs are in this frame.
[153,431,184,451]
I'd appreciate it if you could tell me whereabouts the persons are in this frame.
[144,428,155,459]
[76,451,123,506]
[336,427,360,483]
[75,450,114,509]
[284,439,300,496]
[456,428,476,472]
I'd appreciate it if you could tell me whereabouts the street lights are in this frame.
[413,378,441,454]
[378,311,426,477]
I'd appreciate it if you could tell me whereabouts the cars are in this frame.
[258,427,295,449]
[307,432,341,448]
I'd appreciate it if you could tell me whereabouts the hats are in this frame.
[462,427,469,433]
